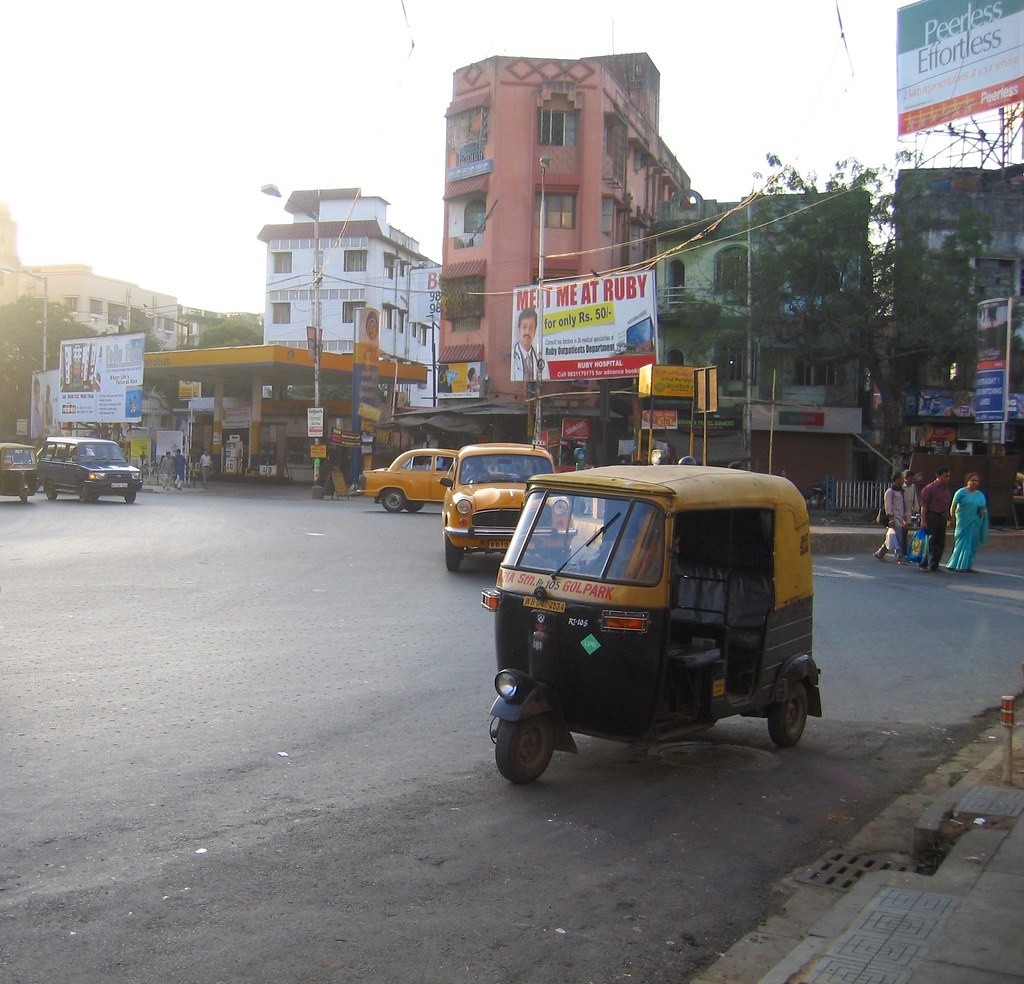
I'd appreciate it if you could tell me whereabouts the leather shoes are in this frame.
[919,564,941,572]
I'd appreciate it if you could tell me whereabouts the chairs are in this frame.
[633,521,775,677]
[463,457,492,485]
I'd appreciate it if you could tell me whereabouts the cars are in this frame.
[445,435,558,575]
[361,448,457,514]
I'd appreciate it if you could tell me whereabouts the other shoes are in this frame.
[949,566,978,573]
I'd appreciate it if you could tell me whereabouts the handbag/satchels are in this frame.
[875,505,889,526]
[906,527,931,569]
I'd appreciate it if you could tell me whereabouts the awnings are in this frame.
[375,413,493,435]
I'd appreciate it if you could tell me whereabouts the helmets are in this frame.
[678,455,697,464]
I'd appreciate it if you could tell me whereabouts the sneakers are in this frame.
[873,553,886,563]
[897,560,909,565]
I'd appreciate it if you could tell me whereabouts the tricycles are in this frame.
[2,442,40,503]
[481,456,827,788]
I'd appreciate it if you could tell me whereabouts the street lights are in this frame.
[260,181,323,483]
[531,154,550,446]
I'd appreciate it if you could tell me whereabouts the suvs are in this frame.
[38,433,143,506]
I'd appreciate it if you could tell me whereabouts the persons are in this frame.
[32,377,45,439]
[70,446,86,463]
[172,449,190,490]
[44,385,54,426]
[198,448,213,489]
[728,461,742,469]
[901,470,920,562]
[780,468,788,478]
[583,507,678,585]
[946,472,990,572]
[918,467,953,572]
[873,471,909,565]
[161,451,173,490]
[583,459,593,469]
[442,458,452,470]
[467,367,480,388]
[462,460,491,484]
[513,307,542,380]
